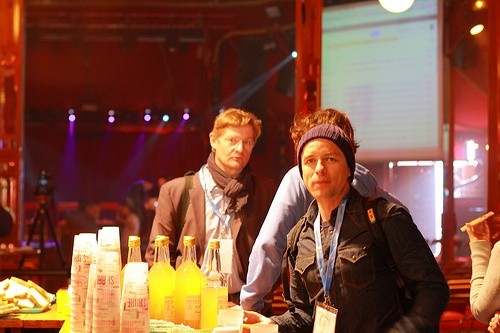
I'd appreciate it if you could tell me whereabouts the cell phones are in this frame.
[460,211,495,231]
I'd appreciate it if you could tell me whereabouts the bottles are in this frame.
[201,239,229,330]
[174,235,201,330]
[147,235,176,324]
[120,234,142,297]
[37,171,47,195]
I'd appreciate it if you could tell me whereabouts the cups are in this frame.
[68,227,151,333]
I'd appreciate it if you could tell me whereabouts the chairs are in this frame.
[22,201,121,240]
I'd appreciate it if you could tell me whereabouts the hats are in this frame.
[296,124,356,184]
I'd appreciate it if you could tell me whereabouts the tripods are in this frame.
[19,203,70,273]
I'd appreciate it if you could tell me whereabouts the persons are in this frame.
[240,109,410,316]
[466,219,500,333]
[145,109,262,308]
[267,124,450,333]
